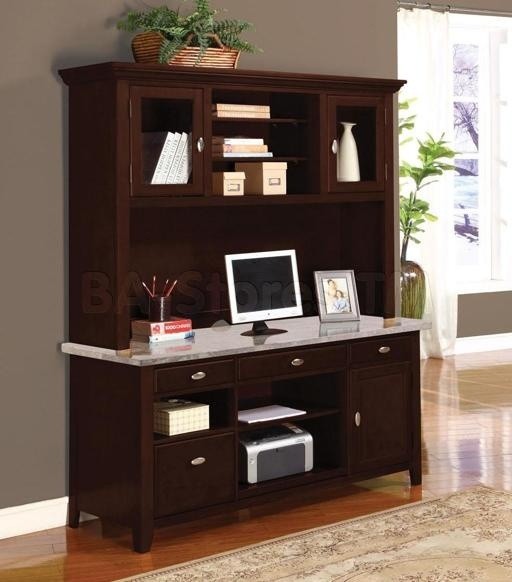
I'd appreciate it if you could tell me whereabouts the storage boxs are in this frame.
[234,161,288,196]
[212,171,247,196]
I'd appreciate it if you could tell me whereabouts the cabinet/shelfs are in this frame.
[50,61,408,347]
[236,342,346,501]
[151,357,236,528]
[349,334,417,477]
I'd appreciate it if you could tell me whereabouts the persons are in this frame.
[328,289,351,313]
[326,278,340,299]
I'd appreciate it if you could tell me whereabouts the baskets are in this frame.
[134,29,242,69]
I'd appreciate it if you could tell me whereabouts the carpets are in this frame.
[102,481,512,582]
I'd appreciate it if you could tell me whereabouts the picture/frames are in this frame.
[313,269,361,324]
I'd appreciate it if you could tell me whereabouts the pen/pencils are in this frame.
[165,279,178,297]
[142,281,153,299]
[152,275,156,298]
[162,279,169,298]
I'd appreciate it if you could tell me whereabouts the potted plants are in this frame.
[398,96,463,319]
[111,0,264,71]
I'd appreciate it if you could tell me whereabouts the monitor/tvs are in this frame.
[223,249,304,336]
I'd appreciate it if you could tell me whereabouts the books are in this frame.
[238,403,308,424]
[211,135,273,158]
[212,103,273,118]
[129,314,196,356]
[140,130,205,186]
